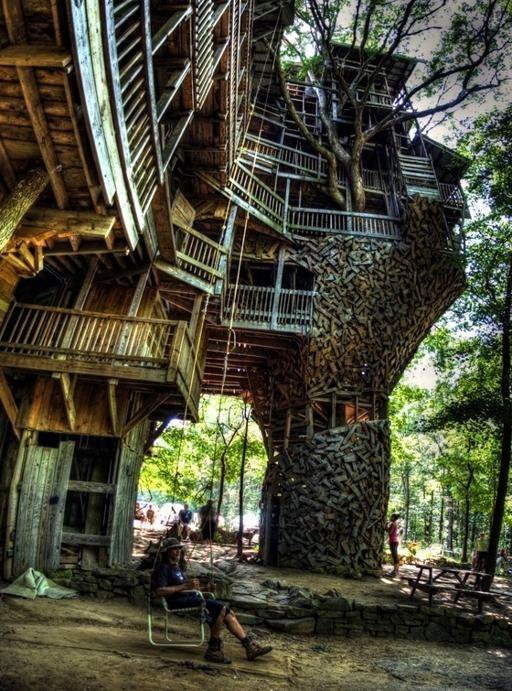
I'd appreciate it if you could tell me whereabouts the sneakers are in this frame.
[204,647,232,665]
[244,641,273,660]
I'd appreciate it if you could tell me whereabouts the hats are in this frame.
[159,536,186,553]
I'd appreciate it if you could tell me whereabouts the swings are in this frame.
[147,1,285,649]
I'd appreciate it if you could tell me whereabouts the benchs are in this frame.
[408,563,501,614]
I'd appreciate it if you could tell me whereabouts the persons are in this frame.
[472,548,478,567]
[385,513,401,577]
[199,499,217,543]
[178,503,192,539]
[146,504,155,526]
[148,536,273,664]
[476,530,488,568]
